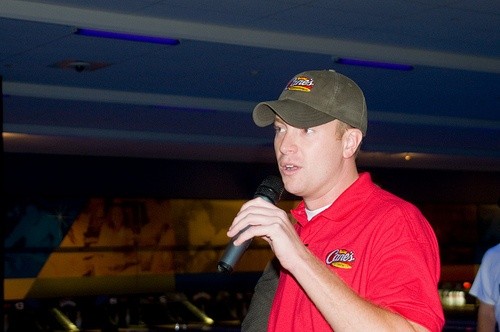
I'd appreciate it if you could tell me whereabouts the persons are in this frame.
[467,241,500,332]
[227,70,446,332]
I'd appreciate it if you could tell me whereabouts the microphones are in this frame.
[216,175,285,273]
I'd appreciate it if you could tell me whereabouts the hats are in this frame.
[252,70,368,139]
